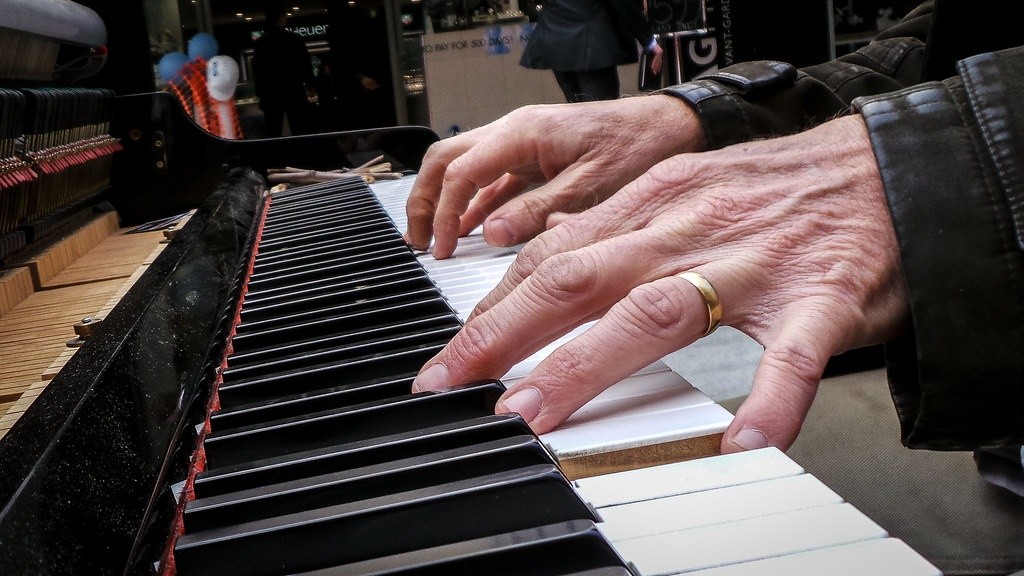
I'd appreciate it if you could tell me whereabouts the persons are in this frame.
[404,0,1024,497]
[251,4,397,138]
[518,0,663,104]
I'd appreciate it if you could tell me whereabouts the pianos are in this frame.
[0,0,948,575]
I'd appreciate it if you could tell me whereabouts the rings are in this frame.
[674,271,721,338]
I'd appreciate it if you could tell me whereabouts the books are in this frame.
[638,50,662,92]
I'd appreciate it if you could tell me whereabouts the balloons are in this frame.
[158,33,220,87]
